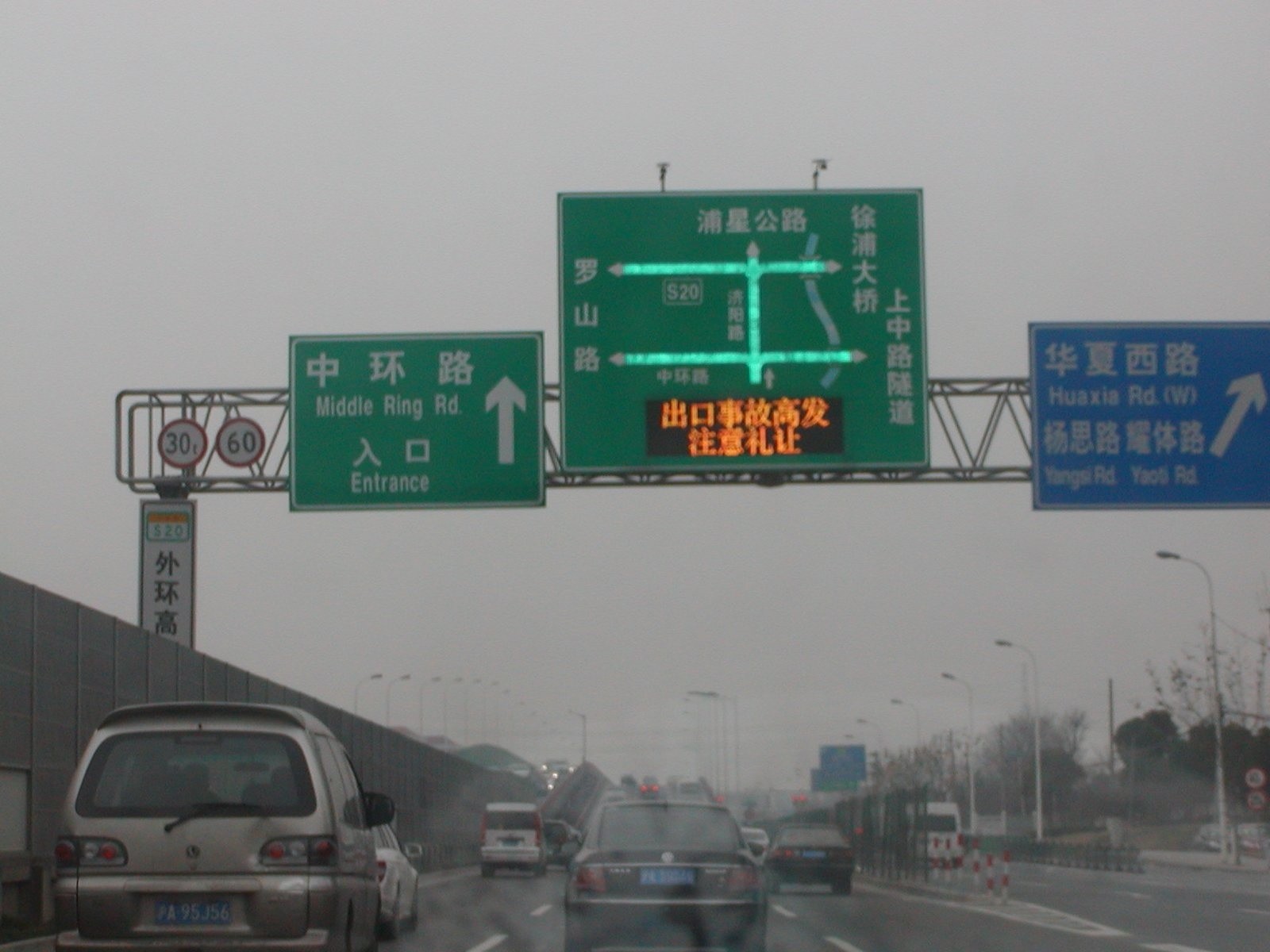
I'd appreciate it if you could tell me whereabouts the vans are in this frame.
[479,801,548,877]
[876,801,961,873]
[51,698,399,952]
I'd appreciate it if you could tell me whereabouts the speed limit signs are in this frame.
[215,417,266,468]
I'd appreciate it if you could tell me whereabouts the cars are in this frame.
[640,782,660,797]
[370,820,421,941]
[740,827,770,857]
[761,822,856,897]
[540,818,586,869]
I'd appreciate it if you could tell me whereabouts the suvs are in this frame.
[544,797,774,952]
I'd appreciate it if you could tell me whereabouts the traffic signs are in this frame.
[1027,319,1270,512]
[291,331,549,511]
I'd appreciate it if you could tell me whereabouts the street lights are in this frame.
[845,697,920,784]
[993,637,1045,847]
[941,671,977,840]
[1155,549,1232,865]
